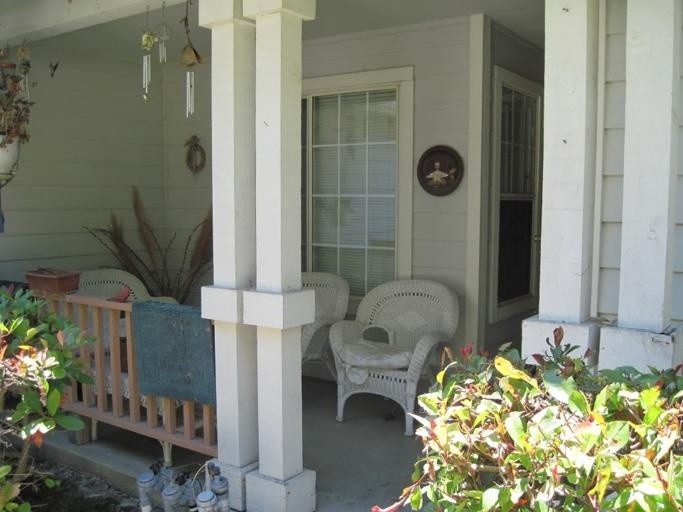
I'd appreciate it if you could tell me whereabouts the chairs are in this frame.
[301,271,461,435]
[46,268,180,357]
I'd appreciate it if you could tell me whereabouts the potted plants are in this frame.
[0,42,39,184]
